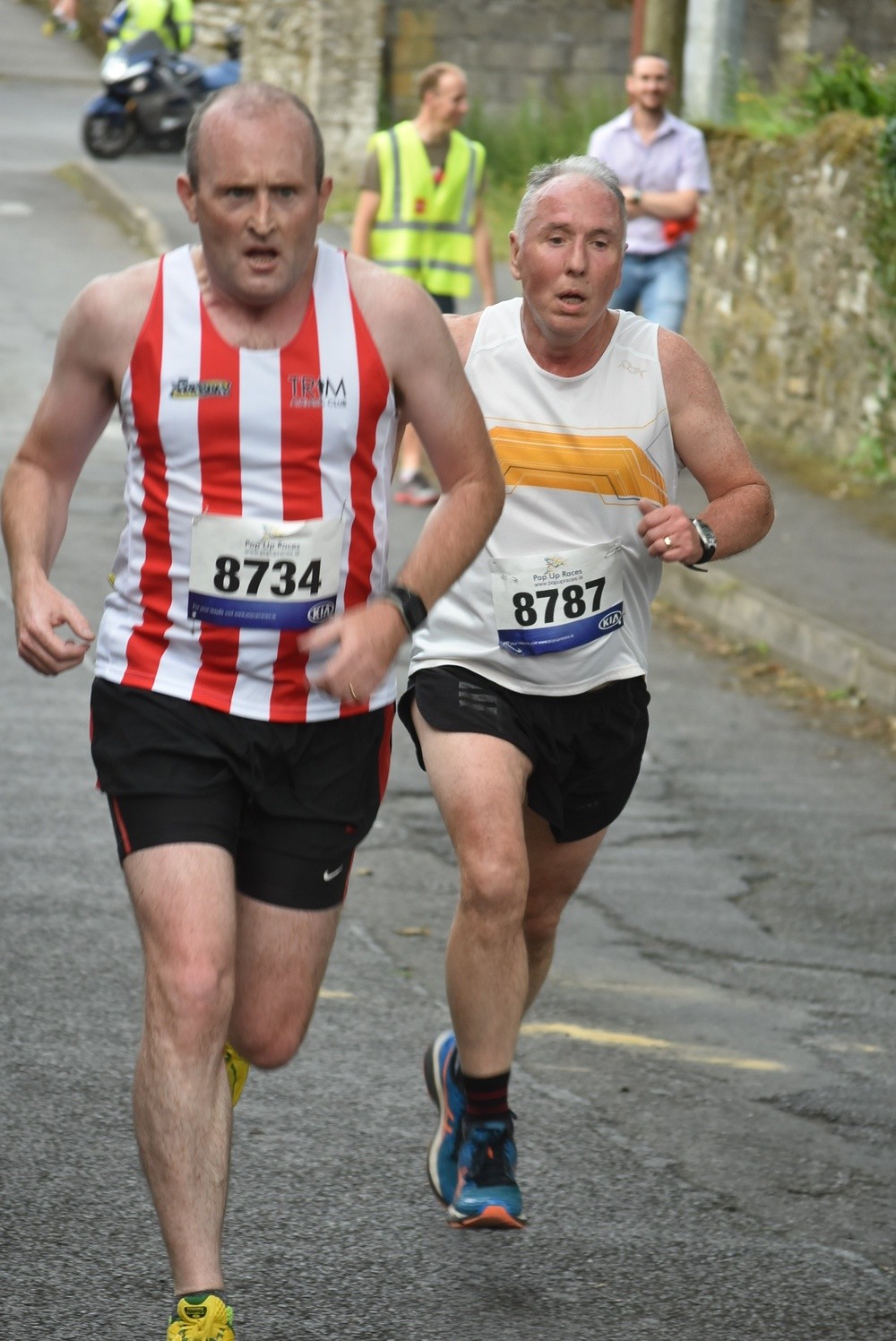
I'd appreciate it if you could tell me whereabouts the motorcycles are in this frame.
[79,0,248,159]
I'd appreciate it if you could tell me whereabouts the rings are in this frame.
[664,535,674,551]
[347,682,362,704]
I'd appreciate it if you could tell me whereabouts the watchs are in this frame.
[378,582,429,636]
[679,516,716,572]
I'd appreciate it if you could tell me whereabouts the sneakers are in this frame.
[224,1041,251,1108]
[392,470,439,505]
[170,1292,239,1341]
[424,1029,526,1228]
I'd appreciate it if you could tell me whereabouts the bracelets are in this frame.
[630,186,642,205]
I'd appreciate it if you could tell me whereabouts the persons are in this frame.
[0,81,507,1341]
[44,0,713,506]
[411,158,777,1230]
